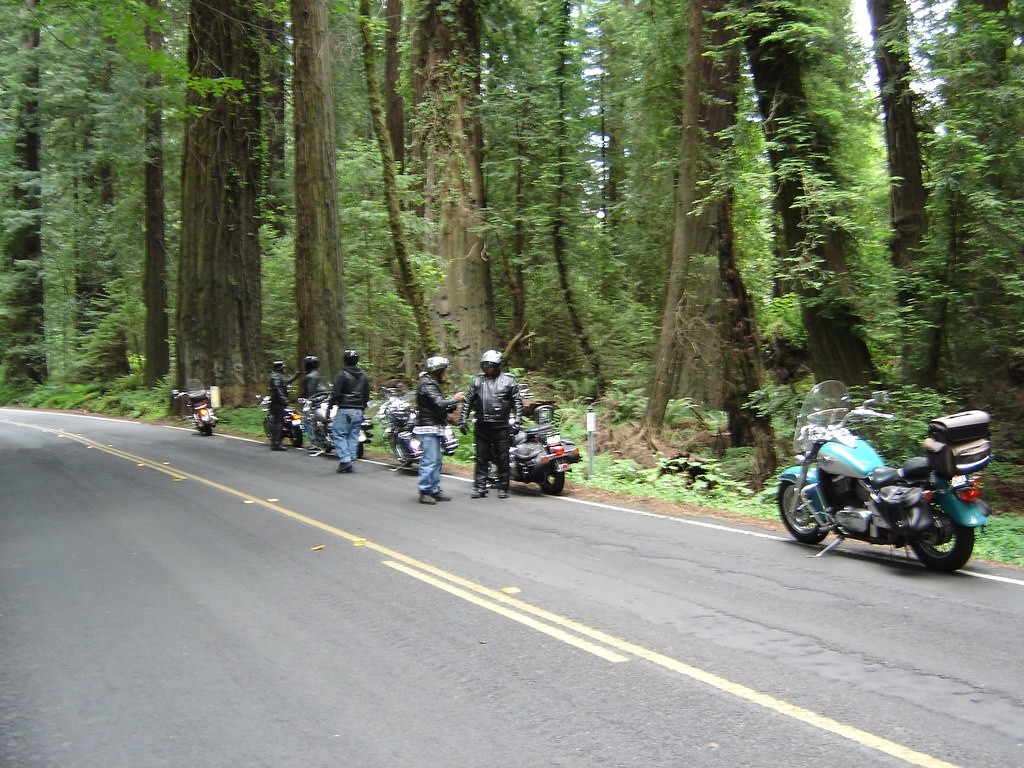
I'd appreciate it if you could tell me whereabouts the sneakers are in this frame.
[337,462,352,472]
[497,488,511,497]
[272,444,286,451]
[470,490,486,497]
[418,493,451,504]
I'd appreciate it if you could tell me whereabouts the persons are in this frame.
[331,350,369,473]
[298,356,321,449]
[459,350,523,498]
[270,361,303,451]
[412,356,465,505]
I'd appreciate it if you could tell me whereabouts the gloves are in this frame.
[459,424,470,436]
[508,423,520,436]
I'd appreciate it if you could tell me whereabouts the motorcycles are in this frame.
[374,386,459,473]
[777,379,994,573]
[468,403,582,496]
[296,375,374,458]
[255,387,306,447]
[170,378,219,437]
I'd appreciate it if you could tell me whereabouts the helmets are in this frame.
[479,349,503,368]
[304,355,319,371]
[273,360,287,370]
[344,349,359,366]
[426,356,449,372]
